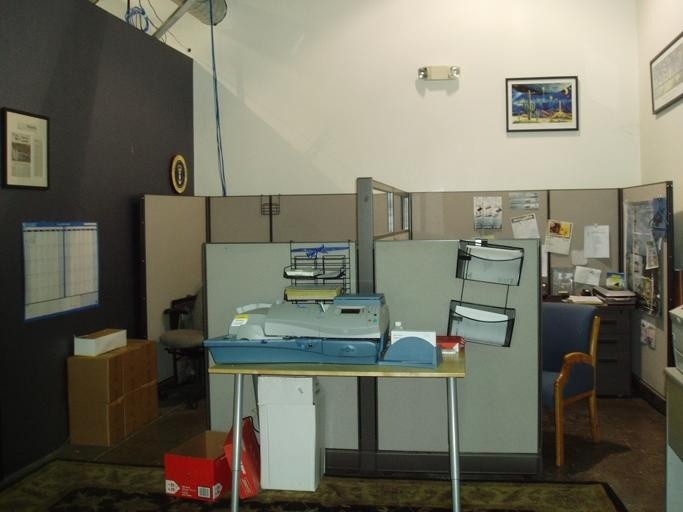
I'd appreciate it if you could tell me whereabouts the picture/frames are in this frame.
[504,74,579,132]
[649,32,683,115]
[1,104,51,191]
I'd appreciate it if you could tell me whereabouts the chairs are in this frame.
[157,280,208,409]
[536,300,605,470]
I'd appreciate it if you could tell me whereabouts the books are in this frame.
[562,284,634,307]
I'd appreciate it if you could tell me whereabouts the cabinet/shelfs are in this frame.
[593,303,636,399]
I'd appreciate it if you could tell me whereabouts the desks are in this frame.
[203,343,471,511]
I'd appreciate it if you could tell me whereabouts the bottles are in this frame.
[390,320,404,332]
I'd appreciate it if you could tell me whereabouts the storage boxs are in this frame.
[63,330,160,452]
[159,418,260,503]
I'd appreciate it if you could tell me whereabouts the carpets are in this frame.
[0,452,631,511]
